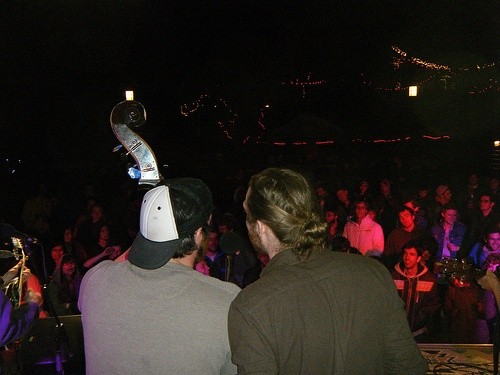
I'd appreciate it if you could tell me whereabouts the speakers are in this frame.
[21,314,86,375]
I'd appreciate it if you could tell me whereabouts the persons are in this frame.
[0,256,43,347]
[79,178,242,375]
[228,168,430,375]
[42,179,500,344]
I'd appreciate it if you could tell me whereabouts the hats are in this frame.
[170,178,216,216]
[128,185,204,270]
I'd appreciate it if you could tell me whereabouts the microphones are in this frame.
[0,222,44,245]
[218,232,243,256]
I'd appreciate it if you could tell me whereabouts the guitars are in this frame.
[433,256,500,285]
[0,236,44,363]
